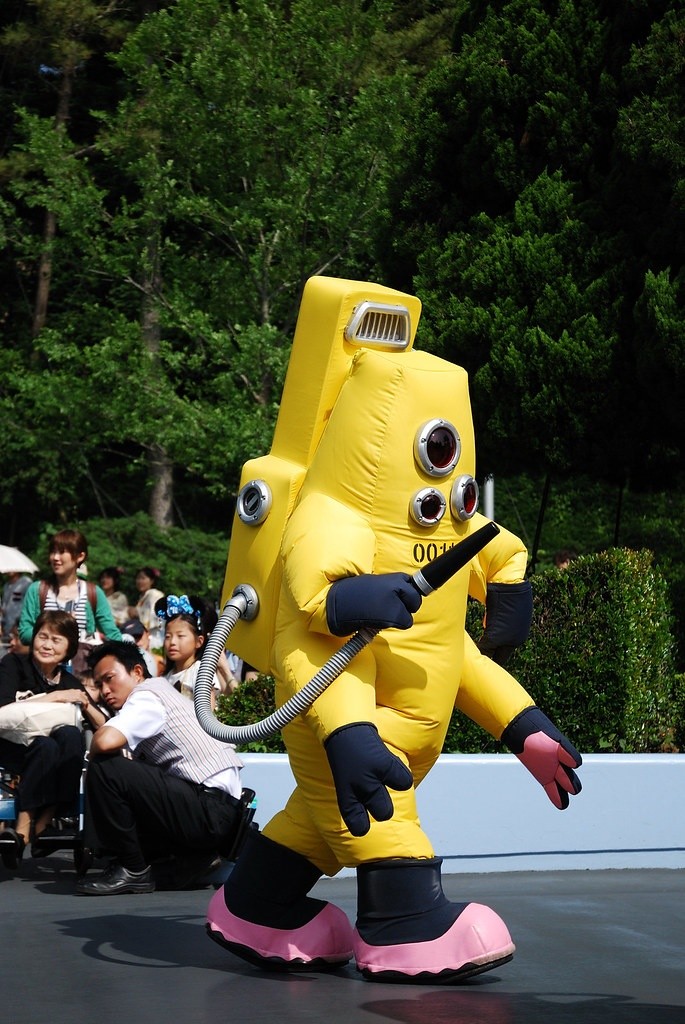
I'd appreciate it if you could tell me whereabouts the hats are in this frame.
[122,618,151,639]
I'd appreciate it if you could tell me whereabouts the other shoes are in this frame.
[0,828,27,869]
[30,826,77,858]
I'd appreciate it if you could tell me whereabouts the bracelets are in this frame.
[228,677,235,685]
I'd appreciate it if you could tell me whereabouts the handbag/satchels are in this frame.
[0,690,91,746]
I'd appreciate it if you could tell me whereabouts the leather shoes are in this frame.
[157,855,233,893]
[74,863,157,897]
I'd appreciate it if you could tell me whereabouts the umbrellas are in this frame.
[0,544,40,574]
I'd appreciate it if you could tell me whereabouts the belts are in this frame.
[204,787,243,809]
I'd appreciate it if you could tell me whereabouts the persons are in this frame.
[0,529,260,894]
